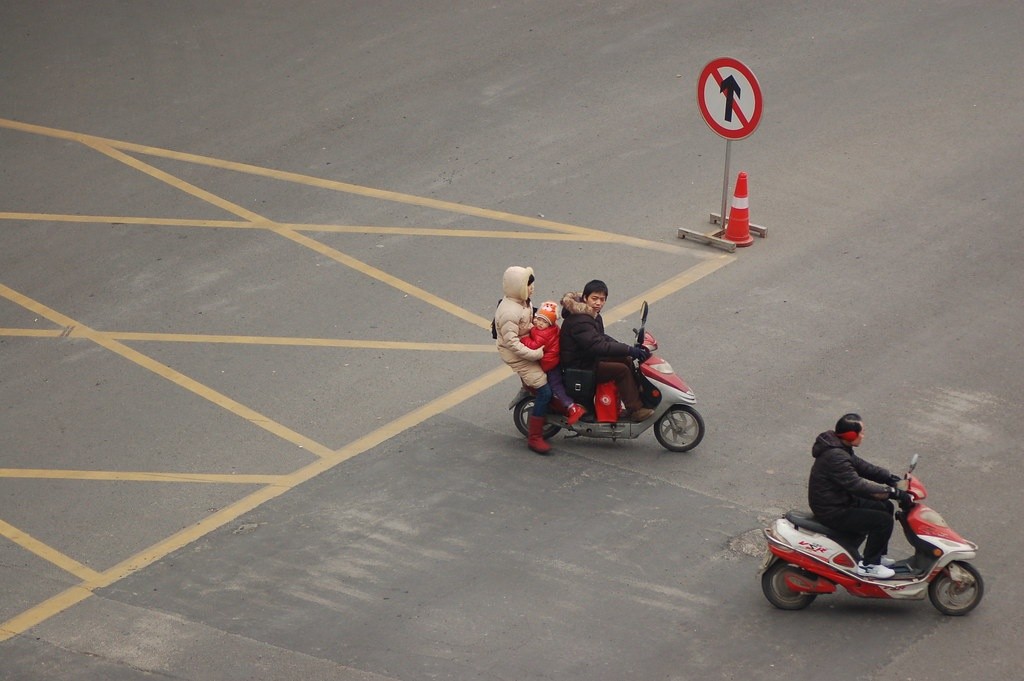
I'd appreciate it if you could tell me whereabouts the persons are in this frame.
[560,280,655,423]
[495,266,586,453]
[808,413,912,579]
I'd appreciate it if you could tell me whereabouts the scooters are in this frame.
[508,300,706,453]
[759,453,985,617]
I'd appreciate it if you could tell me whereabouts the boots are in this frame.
[527,415,552,454]
[566,403,586,426]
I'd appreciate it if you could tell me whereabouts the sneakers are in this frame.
[857,560,896,579]
[880,555,896,567]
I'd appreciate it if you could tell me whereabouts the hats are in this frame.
[536,302,558,326]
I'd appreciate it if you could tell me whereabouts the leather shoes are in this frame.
[625,408,654,421]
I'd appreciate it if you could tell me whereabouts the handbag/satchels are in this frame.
[491,299,539,340]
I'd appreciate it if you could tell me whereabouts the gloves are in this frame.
[885,474,902,486]
[889,486,905,499]
[628,346,649,361]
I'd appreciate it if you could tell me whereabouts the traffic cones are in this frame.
[720,171,754,247]
